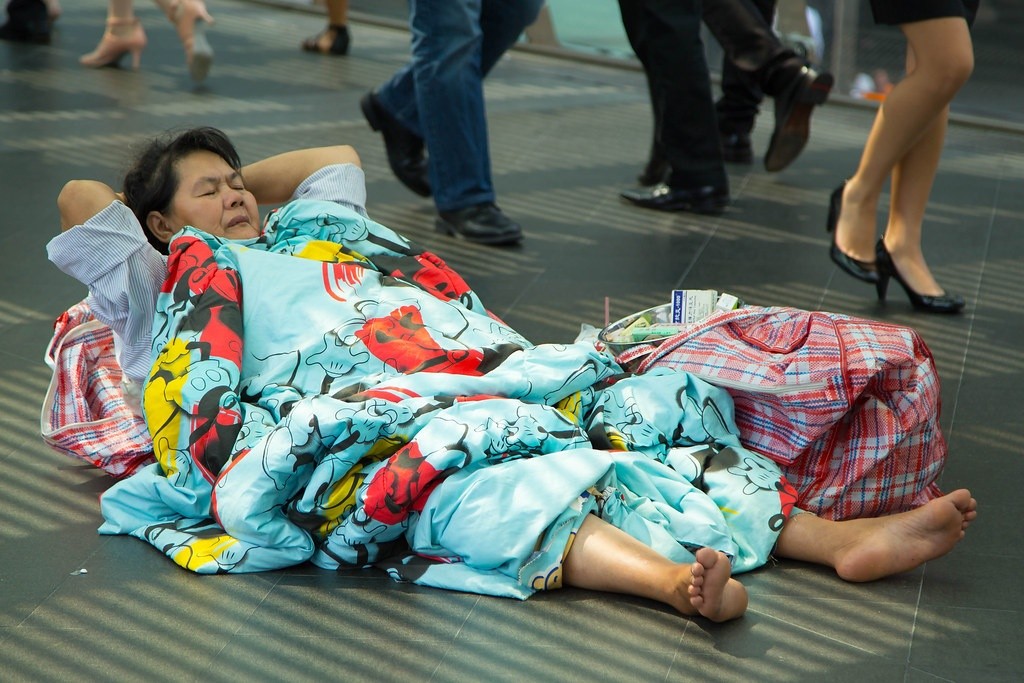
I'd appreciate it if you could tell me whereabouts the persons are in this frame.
[613,0,841,217]
[360,2,545,247]
[300,0,351,57]
[57,123,979,627]
[0,0,54,51]
[80,0,219,81]
[823,0,976,318]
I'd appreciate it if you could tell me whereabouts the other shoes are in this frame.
[719,136,753,165]
[0,24,51,45]
[763,64,835,172]
[303,24,350,55]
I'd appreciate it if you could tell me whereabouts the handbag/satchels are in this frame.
[614,303,950,522]
[39,297,158,480]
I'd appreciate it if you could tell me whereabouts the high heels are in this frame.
[169,0,215,81]
[825,182,879,283]
[874,238,967,314]
[80,19,146,68]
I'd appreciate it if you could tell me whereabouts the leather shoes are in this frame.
[620,182,728,215]
[636,174,659,184]
[435,201,523,242]
[359,90,434,198]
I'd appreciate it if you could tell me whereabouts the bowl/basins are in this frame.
[599,297,745,373]
